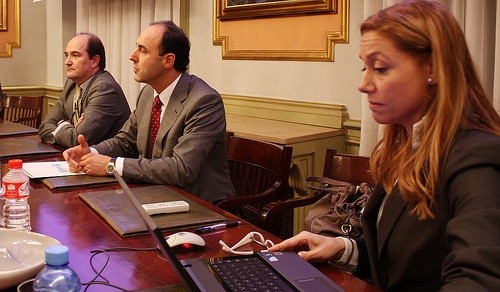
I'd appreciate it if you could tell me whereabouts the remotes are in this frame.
[141,200,189,216]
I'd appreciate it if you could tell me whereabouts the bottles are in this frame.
[32,237,80,292]
[2,159,31,232]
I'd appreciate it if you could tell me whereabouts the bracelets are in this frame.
[344,238,354,265]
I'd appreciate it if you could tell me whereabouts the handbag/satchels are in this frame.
[304,175,372,238]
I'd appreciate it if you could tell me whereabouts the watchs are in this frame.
[106,158,116,176]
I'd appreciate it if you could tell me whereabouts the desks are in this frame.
[0,119,383,292]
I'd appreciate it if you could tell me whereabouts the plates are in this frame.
[0,228,62,290]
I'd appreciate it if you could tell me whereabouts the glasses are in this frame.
[219,231,274,255]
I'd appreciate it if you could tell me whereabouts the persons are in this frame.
[39,33,131,147]
[63,20,245,219]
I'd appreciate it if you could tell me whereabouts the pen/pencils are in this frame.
[194,221,242,233]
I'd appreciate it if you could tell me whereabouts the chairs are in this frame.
[0,94,43,129]
[259,148,376,234]
[216,131,294,240]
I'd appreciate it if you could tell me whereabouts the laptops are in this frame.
[112,170,344,292]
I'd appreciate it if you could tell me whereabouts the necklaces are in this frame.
[268,0,500,292]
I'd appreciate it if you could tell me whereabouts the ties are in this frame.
[148,95,163,160]
[73,86,83,128]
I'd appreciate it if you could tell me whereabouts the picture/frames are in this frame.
[0,0,8,31]
[215,0,338,21]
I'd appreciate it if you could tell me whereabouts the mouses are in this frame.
[158,232,206,252]
